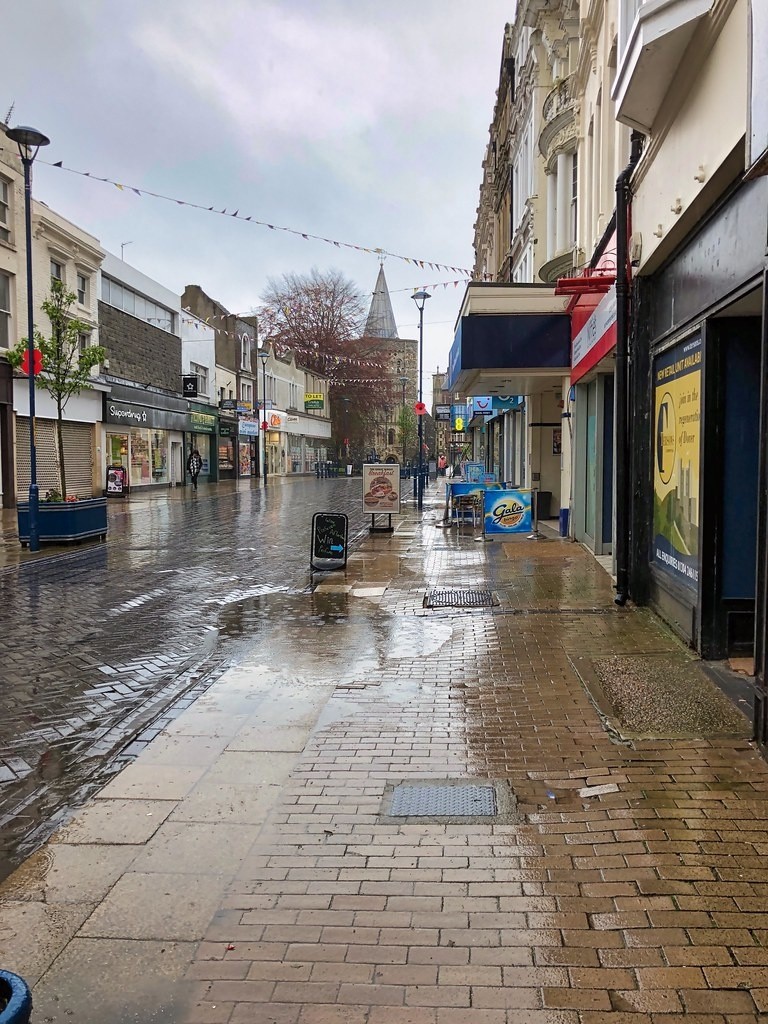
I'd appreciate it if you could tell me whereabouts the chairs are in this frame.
[453,494,477,528]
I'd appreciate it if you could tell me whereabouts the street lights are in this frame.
[411,292,434,509]
[4,126,51,552]
[257,353,270,485]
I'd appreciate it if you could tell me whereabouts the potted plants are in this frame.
[6,281,107,548]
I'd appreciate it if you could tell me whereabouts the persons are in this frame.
[186,450,203,492]
[439,455,446,476]
